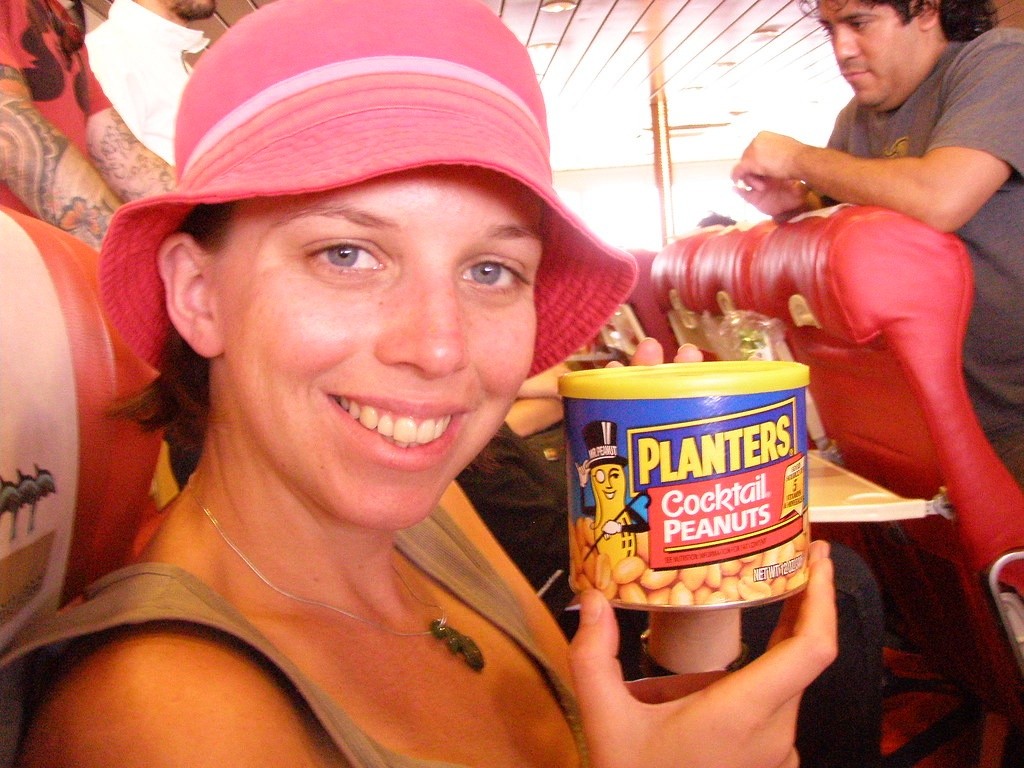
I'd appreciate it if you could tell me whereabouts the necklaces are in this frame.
[185,470,486,673]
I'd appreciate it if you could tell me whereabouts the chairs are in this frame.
[625,202,1024,722]
[0,205,165,768]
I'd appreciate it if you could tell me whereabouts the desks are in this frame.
[524,420,928,524]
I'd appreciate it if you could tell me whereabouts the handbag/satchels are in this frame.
[635,551,986,768]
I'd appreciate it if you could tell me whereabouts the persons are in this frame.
[0,0,840,768]
[731,0,1023,490]
[0,1,217,255]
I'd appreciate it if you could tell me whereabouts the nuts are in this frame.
[569,507,809,605]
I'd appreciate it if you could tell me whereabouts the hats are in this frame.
[98,1,639,381]
